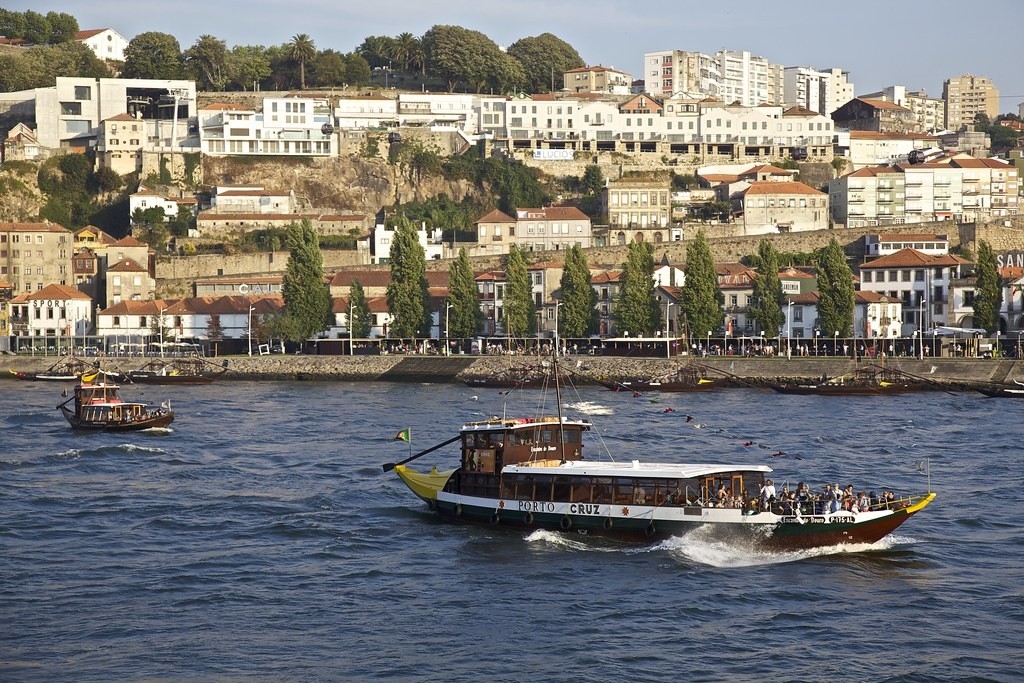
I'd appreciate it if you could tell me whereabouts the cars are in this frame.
[272,345,283,354]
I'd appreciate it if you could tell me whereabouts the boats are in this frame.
[55,335,174,432]
[970,378,1024,397]
[103,335,229,385]
[382,330,936,554]
[9,335,119,382]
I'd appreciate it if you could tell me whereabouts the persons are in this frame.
[860,345,864,356]
[956,344,961,357]
[691,343,809,356]
[877,351,881,359]
[379,339,597,356]
[909,345,913,357]
[888,344,893,356]
[923,345,930,356]
[125,407,164,423]
[61,349,68,356]
[842,343,849,356]
[717,479,894,515]
[823,344,827,356]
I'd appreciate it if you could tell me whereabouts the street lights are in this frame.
[666,303,674,358]
[920,300,927,359]
[446,305,454,356]
[787,301,795,360]
[350,306,357,355]
[160,306,168,357]
[556,303,564,357]
[249,305,256,356]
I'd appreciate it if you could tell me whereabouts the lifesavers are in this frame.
[489,513,500,526]
[453,504,463,517]
[560,516,572,530]
[603,518,612,530]
[644,524,656,538]
[429,499,437,511]
[523,513,534,525]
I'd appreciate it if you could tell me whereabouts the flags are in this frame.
[394,426,411,443]
[161,401,168,409]
[915,460,925,471]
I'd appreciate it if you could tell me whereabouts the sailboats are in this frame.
[461,315,577,389]
[757,308,930,393]
[592,313,728,391]
